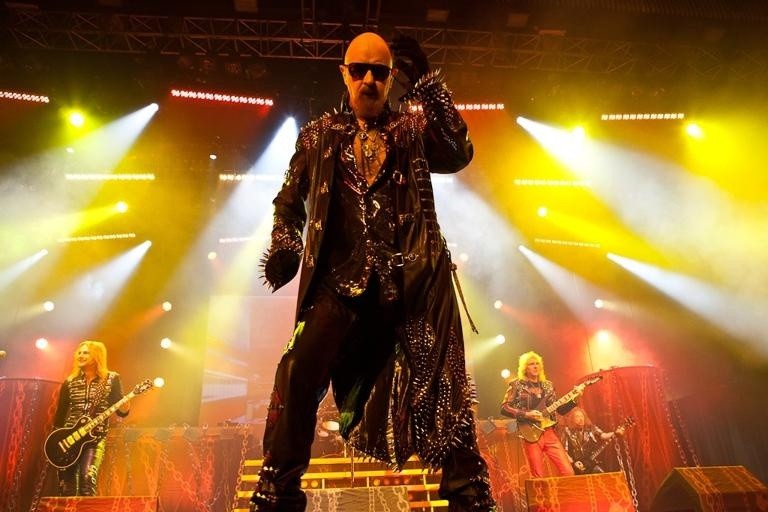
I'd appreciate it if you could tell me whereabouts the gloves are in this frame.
[269,255,294,283]
[392,31,428,85]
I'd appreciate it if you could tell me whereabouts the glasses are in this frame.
[342,63,389,80]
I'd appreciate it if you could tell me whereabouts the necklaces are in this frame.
[528,379,542,399]
[349,121,386,162]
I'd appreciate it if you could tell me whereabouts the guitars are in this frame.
[45,380,152,468]
[517,375,602,443]
[573,417,633,475]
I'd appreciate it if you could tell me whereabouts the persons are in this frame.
[500,351,585,479]
[558,406,625,476]
[51,340,130,497]
[247,32,498,511]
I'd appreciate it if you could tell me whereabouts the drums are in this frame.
[319,406,339,431]
